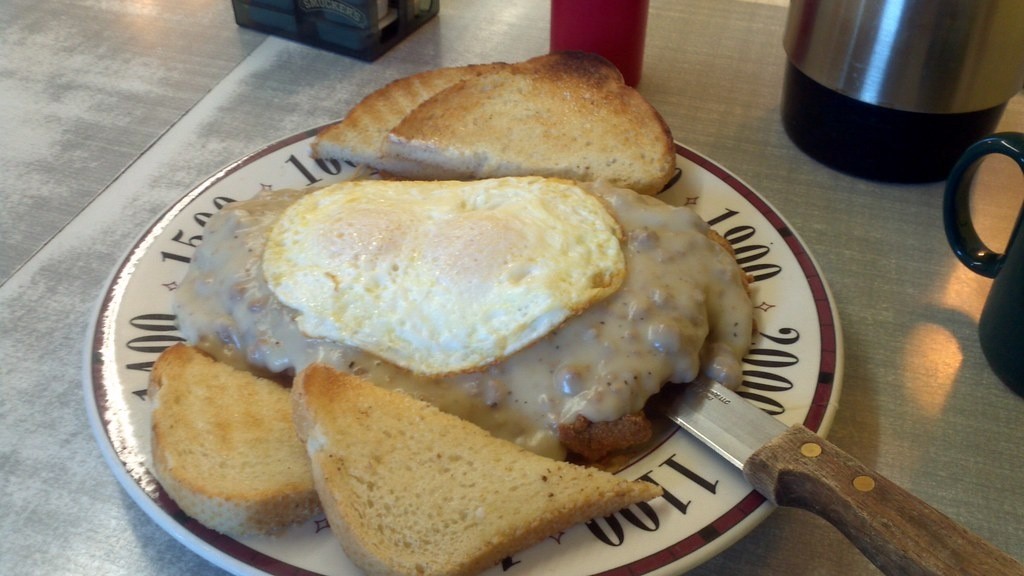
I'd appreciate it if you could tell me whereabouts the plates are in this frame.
[82,117,844,575]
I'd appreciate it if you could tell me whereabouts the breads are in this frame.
[289,362,665,575]
[384,47,678,198]
[145,341,324,539]
[309,60,535,181]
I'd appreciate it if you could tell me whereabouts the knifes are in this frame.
[657,373,1023,575]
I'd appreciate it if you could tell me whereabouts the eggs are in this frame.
[263,178,629,376]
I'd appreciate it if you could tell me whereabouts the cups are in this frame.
[548,0,650,87]
[943,131,1024,397]
